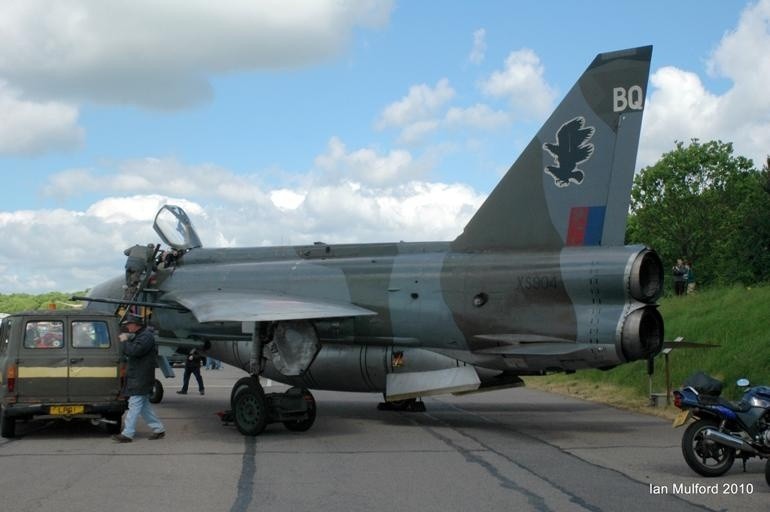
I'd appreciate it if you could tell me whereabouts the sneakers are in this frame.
[175,390,189,395]
[147,432,166,440]
[199,387,207,397]
[111,434,135,444]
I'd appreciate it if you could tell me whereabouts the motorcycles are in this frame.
[669,371,770,487]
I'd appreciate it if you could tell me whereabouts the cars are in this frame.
[169,353,207,367]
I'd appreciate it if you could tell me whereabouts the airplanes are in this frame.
[79,43,726,441]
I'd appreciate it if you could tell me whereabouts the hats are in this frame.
[120,314,146,326]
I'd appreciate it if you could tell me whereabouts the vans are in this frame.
[0,306,126,441]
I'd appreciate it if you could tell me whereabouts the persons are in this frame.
[113,314,166,442]
[684,262,696,295]
[28,322,40,346]
[673,258,687,295]
[124,244,158,287]
[176,349,205,394]
